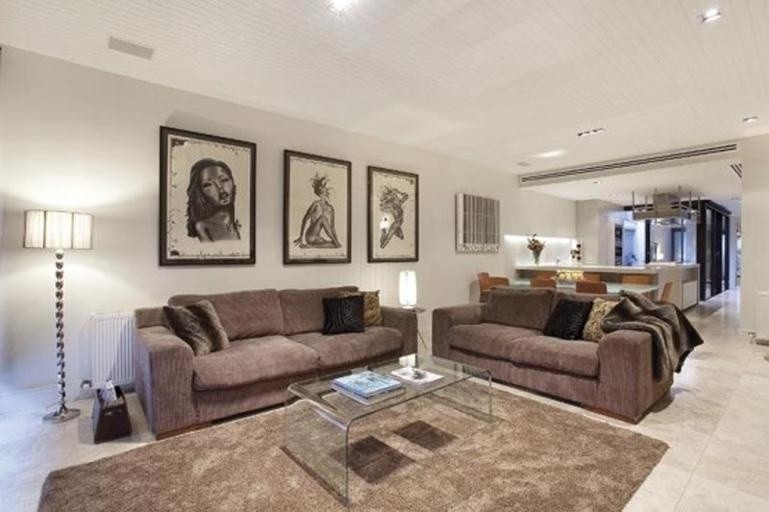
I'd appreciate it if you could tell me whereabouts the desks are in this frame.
[511,280,661,300]
[412,307,426,351]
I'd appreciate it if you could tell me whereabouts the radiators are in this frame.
[92,313,136,390]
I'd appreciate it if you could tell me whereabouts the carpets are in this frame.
[35,369,670,512]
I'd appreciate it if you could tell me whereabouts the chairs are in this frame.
[481,277,509,301]
[659,282,672,301]
[576,281,606,293]
[533,279,557,290]
[477,272,488,291]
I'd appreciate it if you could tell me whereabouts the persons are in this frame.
[293,174,341,248]
[185,159,243,241]
[378,187,408,249]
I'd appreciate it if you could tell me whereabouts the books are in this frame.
[332,369,407,406]
[393,365,443,386]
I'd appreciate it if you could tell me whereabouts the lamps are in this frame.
[23,210,92,423]
[397,271,417,310]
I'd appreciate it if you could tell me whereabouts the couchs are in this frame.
[432,286,673,422]
[135,286,417,437]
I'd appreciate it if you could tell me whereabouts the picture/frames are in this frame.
[283,149,352,263]
[367,167,419,263]
[159,126,257,265]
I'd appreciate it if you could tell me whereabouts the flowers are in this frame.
[530,240,543,253]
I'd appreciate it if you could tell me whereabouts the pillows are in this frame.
[542,300,592,339]
[343,290,383,324]
[581,296,620,340]
[161,301,228,356]
[321,296,364,334]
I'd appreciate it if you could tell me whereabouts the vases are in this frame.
[534,254,538,265]
